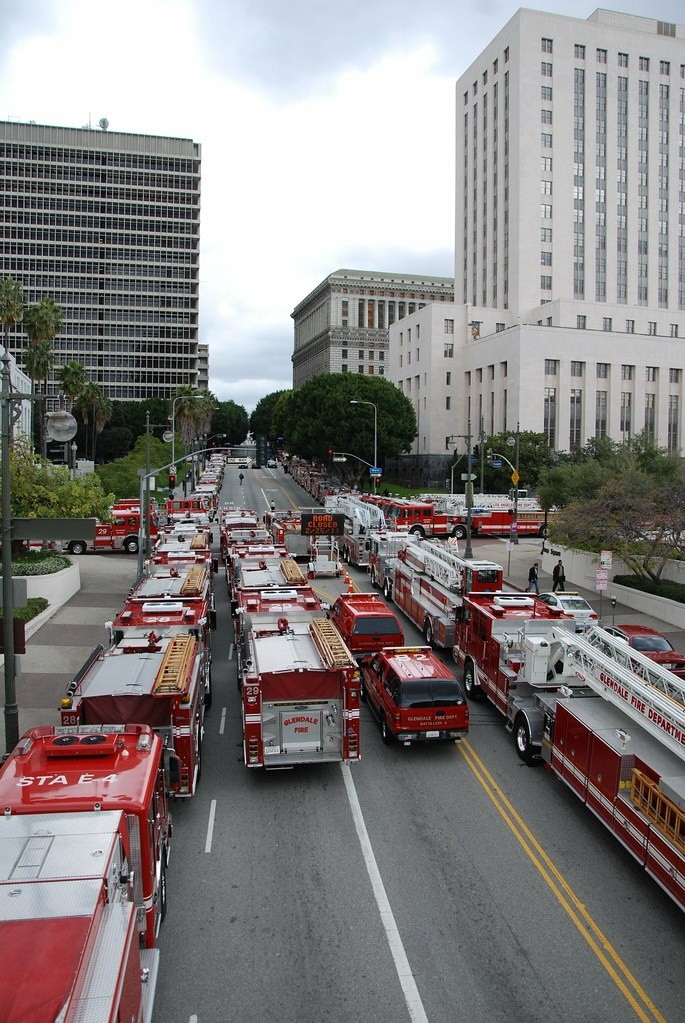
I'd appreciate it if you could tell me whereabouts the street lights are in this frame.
[447,418,475,558]
[204,433,226,470]
[71,441,78,481]
[507,422,519,545]
[142,410,174,559]
[0,349,78,764]
[172,395,204,502]
[349,400,378,496]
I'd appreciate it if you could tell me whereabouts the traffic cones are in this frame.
[344,571,355,593]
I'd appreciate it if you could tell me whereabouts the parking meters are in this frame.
[609,594,617,626]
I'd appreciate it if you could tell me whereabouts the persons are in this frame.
[239,473,244,485]
[383,489,390,497]
[551,560,565,592]
[270,500,276,512]
[524,563,540,594]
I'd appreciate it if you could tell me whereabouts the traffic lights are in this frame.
[327,449,333,455]
[485,448,492,466]
[376,478,380,487]
[169,476,175,489]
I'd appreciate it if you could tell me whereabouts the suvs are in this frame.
[361,646,469,746]
[327,592,404,659]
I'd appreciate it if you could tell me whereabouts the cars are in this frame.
[539,591,685,680]
[188,453,225,500]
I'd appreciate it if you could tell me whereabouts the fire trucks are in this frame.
[324,495,503,648]
[220,507,360,771]
[453,591,685,913]
[60,499,218,802]
[283,453,361,504]
[0,725,179,1023]
[360,494,561,539]
